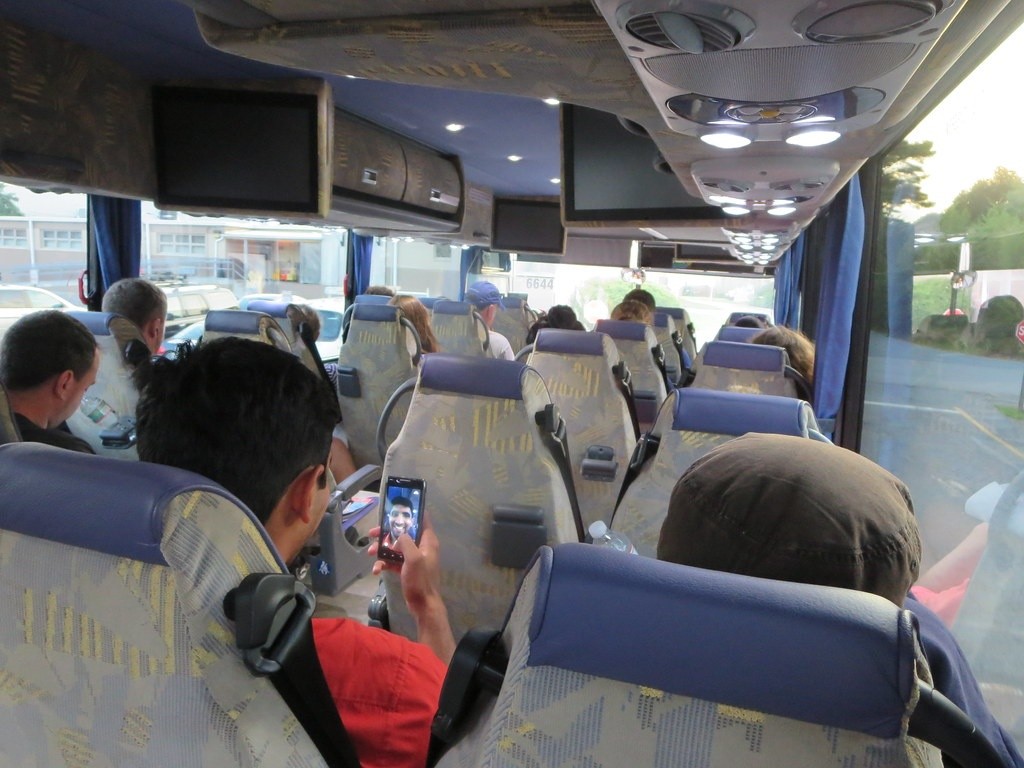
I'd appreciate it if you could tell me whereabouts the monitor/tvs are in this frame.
[490,103,778,267]
[148,77,333,222]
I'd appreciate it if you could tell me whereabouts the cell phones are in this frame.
[377,477,427,564]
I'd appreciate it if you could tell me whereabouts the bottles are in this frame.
[80,395,118,429]
[589,521,638,555]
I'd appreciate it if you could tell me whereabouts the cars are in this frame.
[144,275,345,361]
[0,282,88,336]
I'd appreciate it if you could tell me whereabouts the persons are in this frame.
[735,316,762,330]
[656,428,1024,768]
[365,287,445,363]
[464,281,517,361]
[748,325,816,403]
[526,304,587,351]
[293,305,341,391]
[383,497,413,553]
[0,309,101,455]
[137,339,455,768]
[907,483,1024,627]
[103,275,167,358]
[609,288,693,389]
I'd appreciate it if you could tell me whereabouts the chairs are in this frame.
[0,292,1008,768]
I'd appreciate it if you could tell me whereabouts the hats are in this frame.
[464,281,505,311]
[653,431,922,604]
